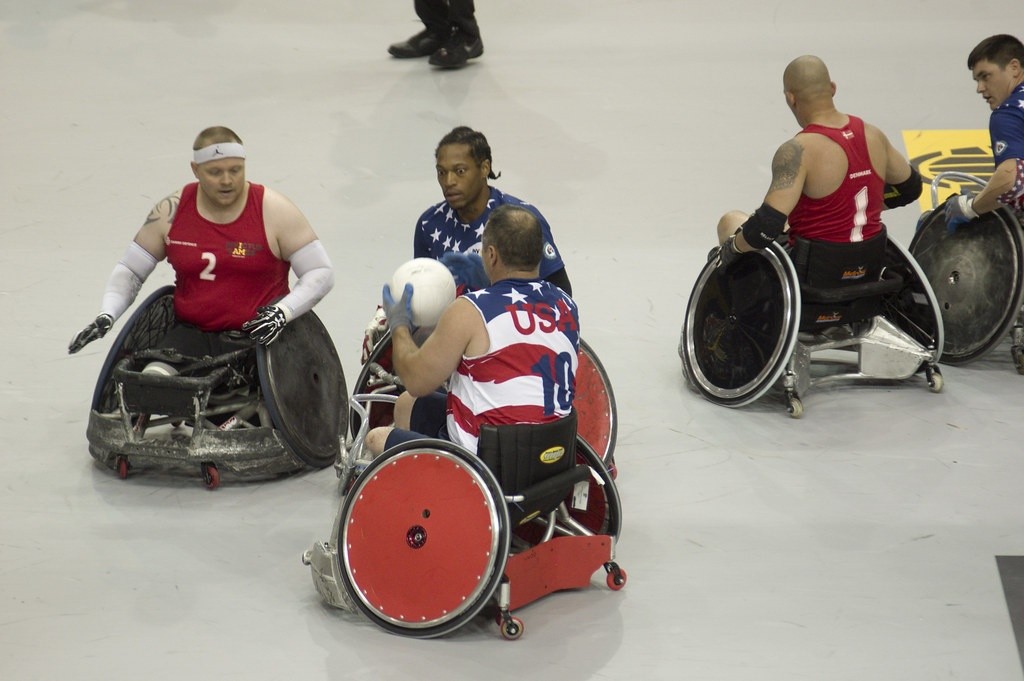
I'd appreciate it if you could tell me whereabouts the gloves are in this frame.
[943,189,980,232]
[69,317,111,353]
[361,308,388,364]
[715,239,736,270]
[382,283,413,335]
[439,252,490,290]
[243,305,286,346]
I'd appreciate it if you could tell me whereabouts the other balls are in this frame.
[390,257,457,328]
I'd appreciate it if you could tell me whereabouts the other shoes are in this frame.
[388,27,452,59]
[429,31,483,67]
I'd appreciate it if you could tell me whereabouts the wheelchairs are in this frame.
[678,227,946,412]
[911,173,1024,375]
[302,407,627,637]
[335,324,619,493]
[83,285,349,489]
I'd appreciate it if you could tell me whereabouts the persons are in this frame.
[916,34,1024,235]
[388,0,484,71]
[302,206,580,565]
[708,54,922,266]
[68,125,335,375]
[361,126,572,395]
